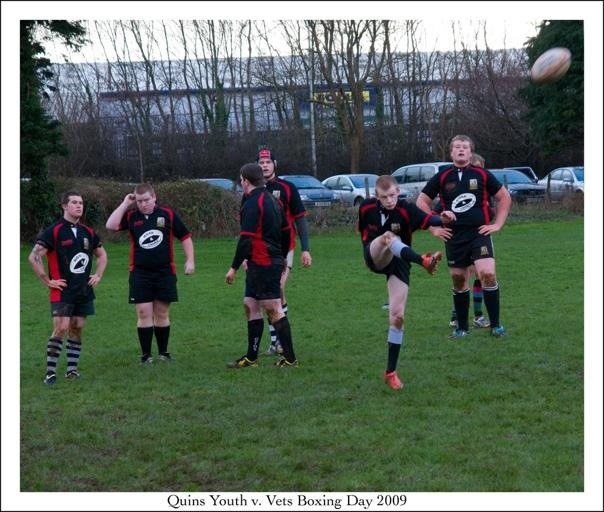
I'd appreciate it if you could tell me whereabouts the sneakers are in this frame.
[64,371,83,380]
[449,314,511,341]
[226,355,260,369]
[267,342,299,368]
[156,352,172,363]
[421,251,443,276]
[383,369,404,392]
[43,376,59,387]
[140,353,156,367]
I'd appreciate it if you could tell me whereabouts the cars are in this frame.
[188,164,583,211]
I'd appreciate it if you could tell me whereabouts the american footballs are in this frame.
[530,46,572,82]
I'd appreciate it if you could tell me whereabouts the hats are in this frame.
[256,148,278,160]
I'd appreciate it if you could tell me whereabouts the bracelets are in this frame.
[96,274,103,278]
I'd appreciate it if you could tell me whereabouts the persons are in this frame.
[358,174,456,390]
[416,134,512,340]
[243,146,312,356]
[432,154,496,328]
[27,192,107,385]
[224,164,298,369]
[104,183,195,365]
